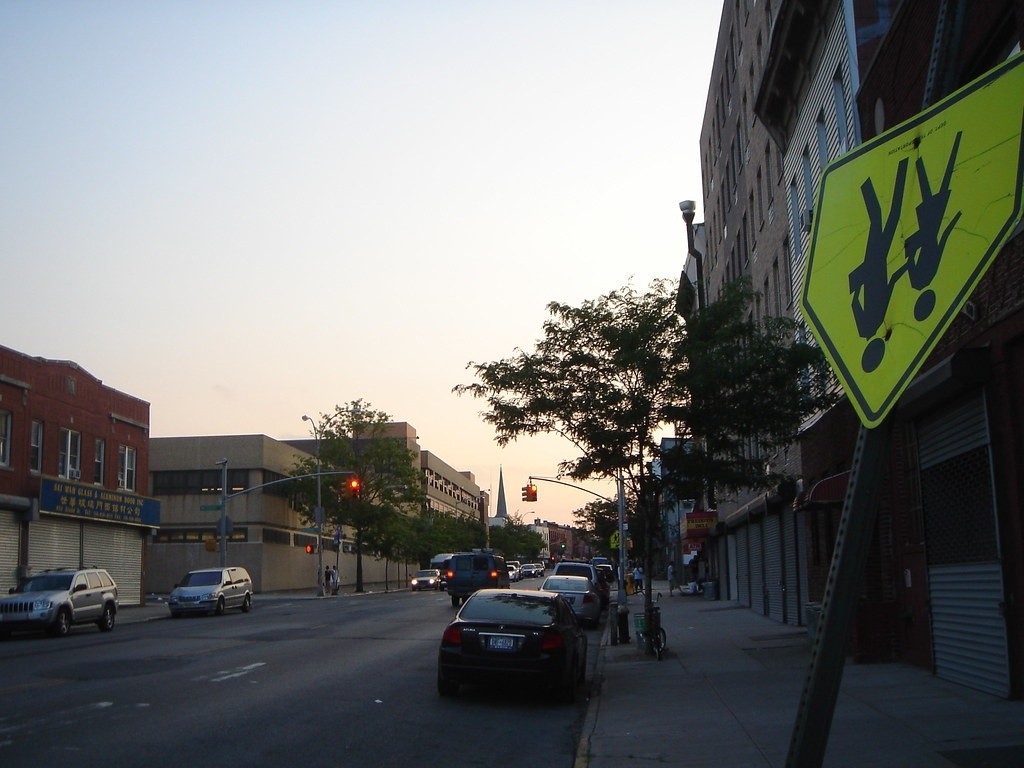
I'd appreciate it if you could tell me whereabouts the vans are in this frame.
[549,557,610,611]
[446,552,510,606]
[430,553,458,590]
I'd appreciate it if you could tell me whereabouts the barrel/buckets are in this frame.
[634,612,645,632]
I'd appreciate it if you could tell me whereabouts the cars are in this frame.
[536,575,604,629]
[505,561,545,583]
[408,569,441,592]
[438,588,587,709]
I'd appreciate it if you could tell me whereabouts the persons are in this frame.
[325,565,340,593]
[668,561,677,596]
[689,556,699,580]
[633,563,643,589]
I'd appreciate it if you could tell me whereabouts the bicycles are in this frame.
[645,590,666,661]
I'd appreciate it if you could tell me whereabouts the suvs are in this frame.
[0,565,120,637]
[169,567,254,615]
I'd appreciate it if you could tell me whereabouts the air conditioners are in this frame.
[118,479,125,487]
[68,469,82,480]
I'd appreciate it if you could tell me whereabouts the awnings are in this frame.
[793,470,850,512]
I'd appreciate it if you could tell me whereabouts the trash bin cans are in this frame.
[702,582,715,599]
[805,601,822,641]
[635,612,661,650]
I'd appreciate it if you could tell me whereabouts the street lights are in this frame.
[520,511,536,540]
[302,409,363,597]
[454,494,486,553]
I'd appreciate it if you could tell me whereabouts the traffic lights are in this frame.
[530,485,538,502]
[561,545,565,551]
[350,480,360,494]
[305,546,315,553]
[521,484,530,502]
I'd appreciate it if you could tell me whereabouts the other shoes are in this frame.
[670,595,673,597]
[680,593,685,596]
